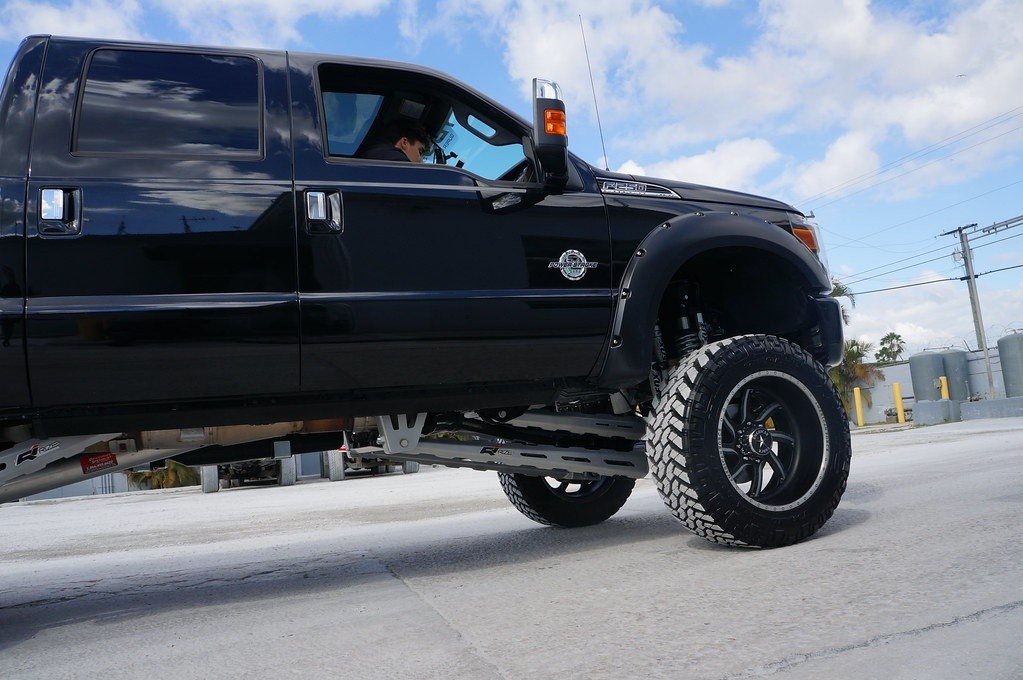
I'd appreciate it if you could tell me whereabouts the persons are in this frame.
[363,126,429,165]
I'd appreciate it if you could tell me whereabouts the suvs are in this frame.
[0,36,850,550]
